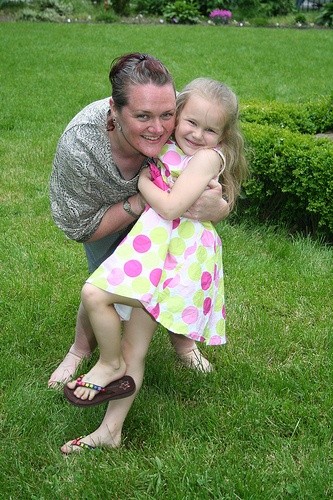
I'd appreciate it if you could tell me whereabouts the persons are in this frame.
[57,77,246,456]
[44,50,231,388]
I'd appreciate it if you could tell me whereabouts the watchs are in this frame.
[123,198,140,219]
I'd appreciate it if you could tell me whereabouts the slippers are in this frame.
[59,435,100,457]
[64,373,136,406]
